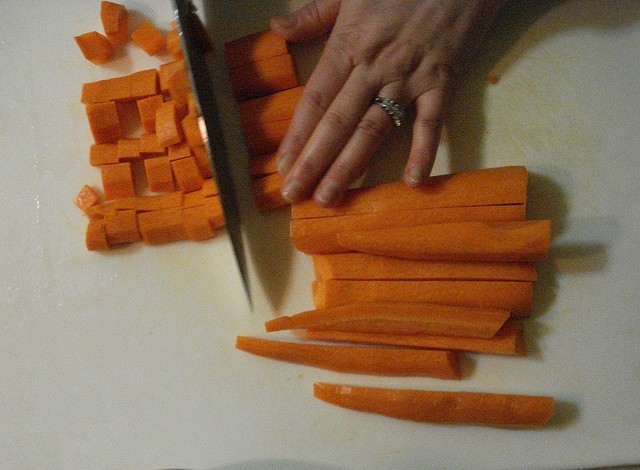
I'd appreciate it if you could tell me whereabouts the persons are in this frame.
[269,1,509,208]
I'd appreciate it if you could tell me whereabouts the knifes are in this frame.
[170,1,254,316]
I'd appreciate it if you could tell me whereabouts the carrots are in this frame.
[314,382,555,428]
[236,166,553,378]
[223,1,327,252]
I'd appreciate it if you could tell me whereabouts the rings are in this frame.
[373,97,408,128]
